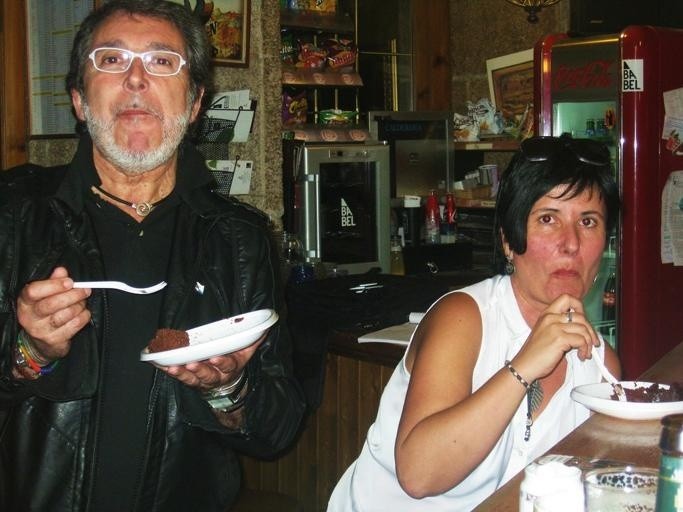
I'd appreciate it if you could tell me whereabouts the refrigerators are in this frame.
[532,25,683,381]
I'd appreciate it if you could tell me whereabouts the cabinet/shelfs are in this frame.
[279,1,364,144]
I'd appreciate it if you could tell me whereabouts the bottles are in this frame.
[584,114,618,141]
[658,414,683,512]
[600,267,617,336]
[421,188,458,245]
[272,232,338,285]
[389,233,405,278]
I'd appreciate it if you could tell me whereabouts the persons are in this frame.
[0,1,309,512]
[327,135,622,512]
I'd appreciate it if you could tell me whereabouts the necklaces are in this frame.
[95,186,176,216]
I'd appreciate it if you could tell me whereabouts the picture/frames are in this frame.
[184,0,249,68]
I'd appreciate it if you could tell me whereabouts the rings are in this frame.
[567,312,572,322]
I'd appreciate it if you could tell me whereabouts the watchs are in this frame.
[200,370,248,409]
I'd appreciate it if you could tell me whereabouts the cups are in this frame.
[582,466,660,511]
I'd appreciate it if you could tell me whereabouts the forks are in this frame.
[70,279,168,295]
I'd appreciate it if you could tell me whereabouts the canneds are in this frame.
[479,164,499,186]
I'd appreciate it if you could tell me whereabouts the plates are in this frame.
[571,381,683,422]
[139,306,279,368]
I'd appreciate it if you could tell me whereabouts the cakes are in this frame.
[146,328,189,353]
[611,379,683,403]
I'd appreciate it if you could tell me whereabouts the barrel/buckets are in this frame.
[577,249,617,336]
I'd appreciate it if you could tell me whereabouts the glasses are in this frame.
[519,133,614,168]
[89,46,187,77]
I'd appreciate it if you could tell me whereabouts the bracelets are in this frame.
[200,369,245,399]
[13,330,59,381]
[220,400,246,413]
[505,360,532,441]
